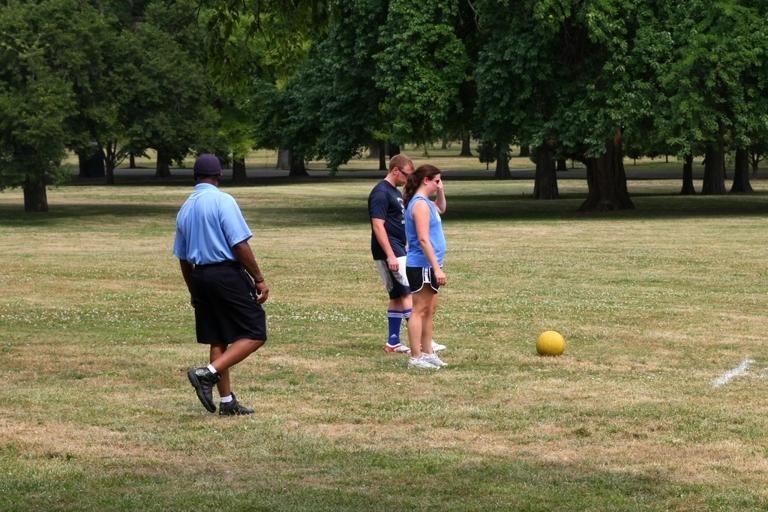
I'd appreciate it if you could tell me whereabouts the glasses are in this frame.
[398,167,409,177]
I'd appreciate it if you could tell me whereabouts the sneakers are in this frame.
[186,366,219,413]
[428,340,446,351]
[422,352,447,367]
[408,357,440,371]
[216,393,255,414]
[384,343,411,354]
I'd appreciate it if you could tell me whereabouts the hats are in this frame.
[195,155,222,177]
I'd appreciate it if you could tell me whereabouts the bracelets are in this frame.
[254,277,265,283]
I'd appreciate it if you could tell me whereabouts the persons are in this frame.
[367,154,450,351]
[171,152,270,417]
[397,163,450,372]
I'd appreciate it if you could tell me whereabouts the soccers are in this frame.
[536,331,565,356]
